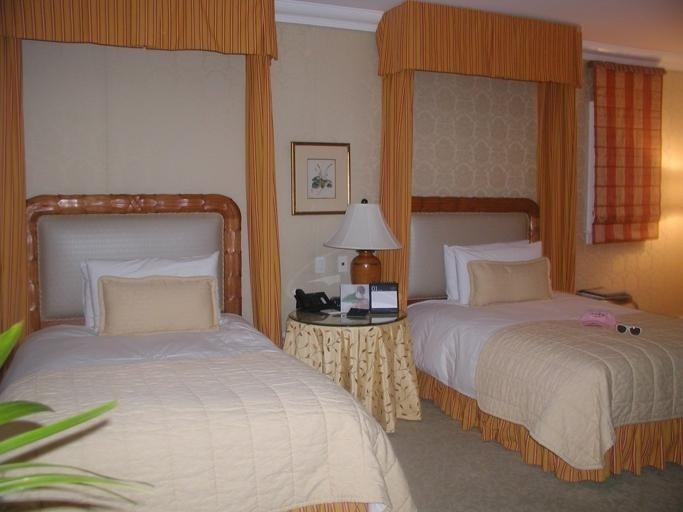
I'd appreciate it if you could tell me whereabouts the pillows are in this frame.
[442,240,553,308]
[80,252,222,335]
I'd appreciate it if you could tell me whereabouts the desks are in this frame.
[284,310,421,434]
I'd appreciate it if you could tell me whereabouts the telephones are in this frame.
[294,289,331,312]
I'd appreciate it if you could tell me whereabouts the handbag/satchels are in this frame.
[294,288,337,312]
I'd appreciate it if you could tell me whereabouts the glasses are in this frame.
[616,324,640,336]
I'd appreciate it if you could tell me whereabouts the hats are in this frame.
[581,311,615,326]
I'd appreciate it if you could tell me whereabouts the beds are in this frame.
[406,198,683,486]
[1,194,372,511]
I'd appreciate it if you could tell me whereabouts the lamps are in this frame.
[324,198,402,285]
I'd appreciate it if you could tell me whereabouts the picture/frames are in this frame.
[291,141,351,215]
